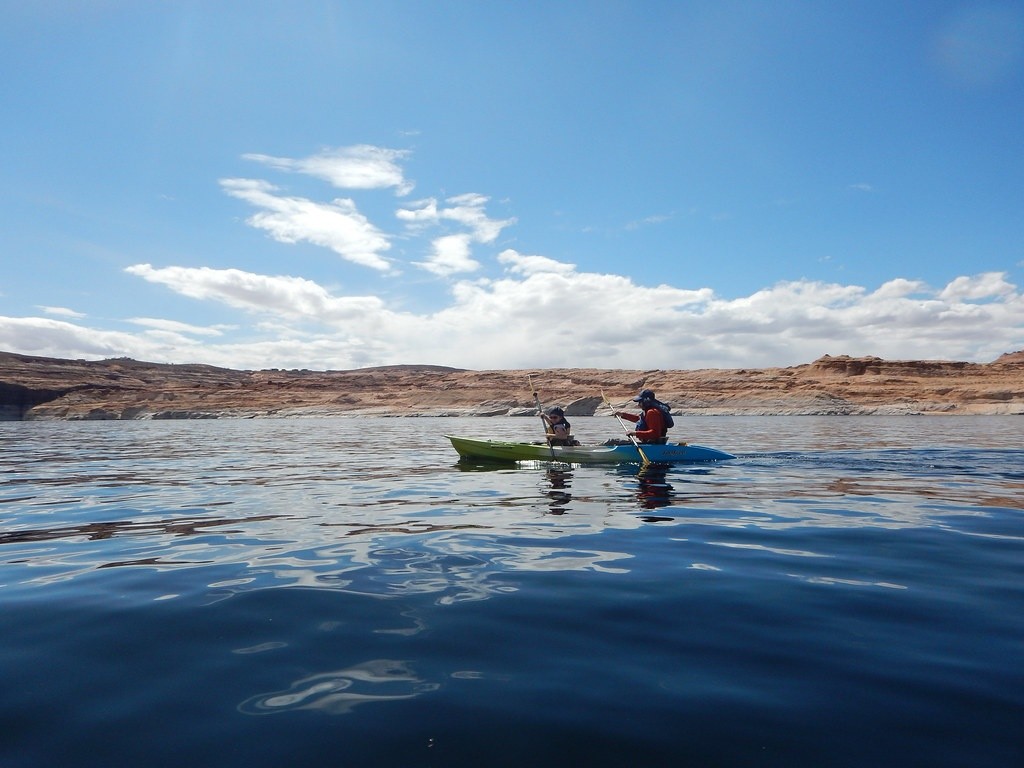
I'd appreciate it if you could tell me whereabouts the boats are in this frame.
[443,434,737,462]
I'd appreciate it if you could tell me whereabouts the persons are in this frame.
[540,406,571,445]
[604,389,674,447]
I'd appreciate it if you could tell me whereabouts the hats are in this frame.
[546,406,564,417]
[633,388,656,403]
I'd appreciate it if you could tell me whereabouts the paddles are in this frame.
[528,375,558,464]
[600,388,651,465]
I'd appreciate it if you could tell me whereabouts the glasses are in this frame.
[549,416,559,420]
